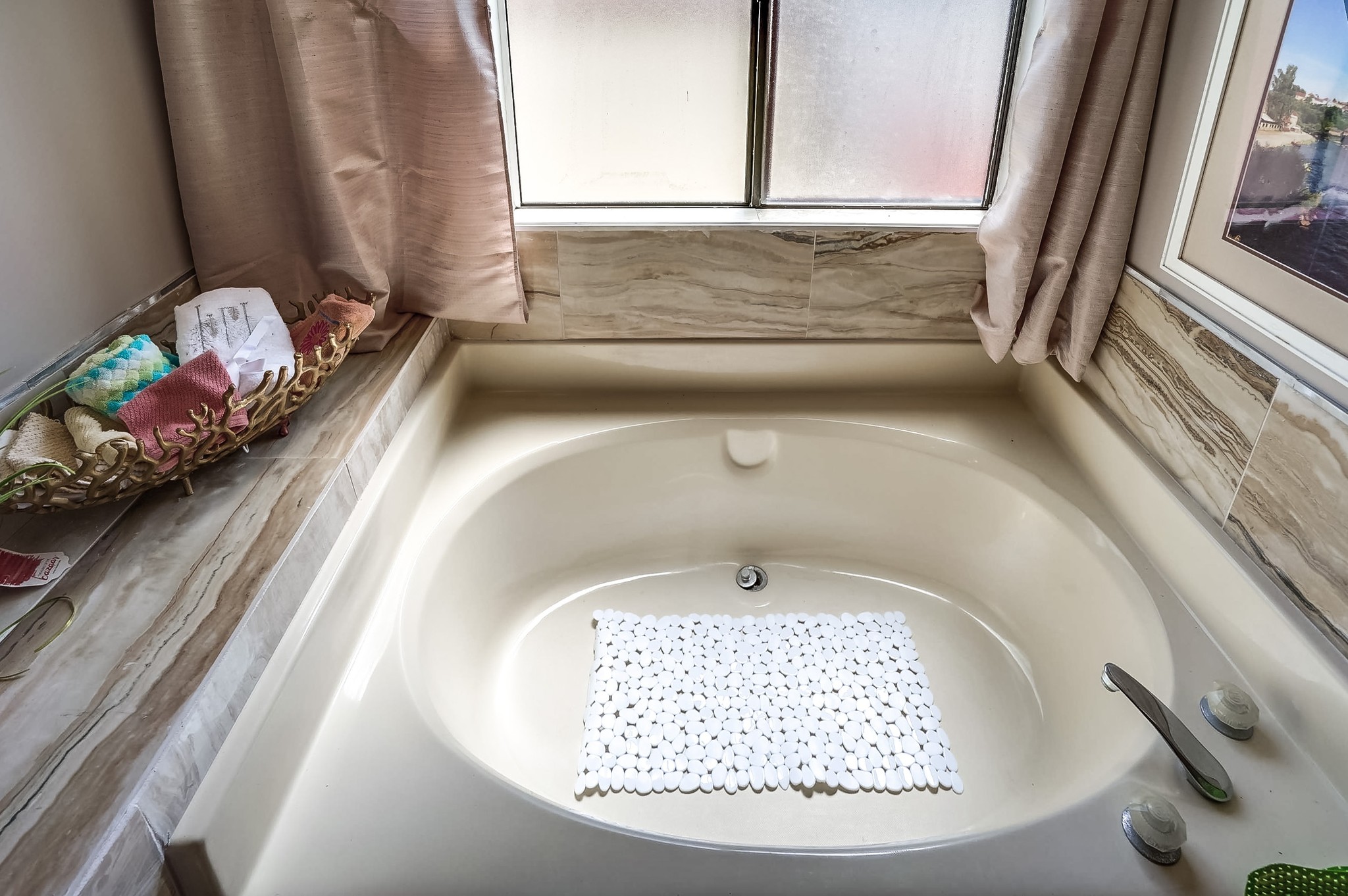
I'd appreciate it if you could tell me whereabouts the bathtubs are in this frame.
[397,406,1181,867]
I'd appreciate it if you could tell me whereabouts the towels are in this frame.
[113,346,248,474]
[289,293,376,389]
[175,285,298,412]
[62,332,173,424]
[0,405,152,511]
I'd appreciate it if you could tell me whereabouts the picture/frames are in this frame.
[1160,0,1348,389]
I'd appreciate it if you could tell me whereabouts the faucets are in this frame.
[1101,660,1236,803]
